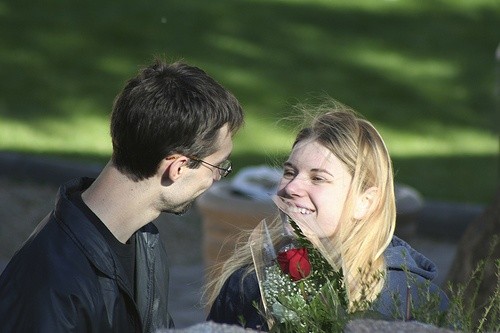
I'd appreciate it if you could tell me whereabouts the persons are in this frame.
[0,58,246,333]
[201,96,460,333]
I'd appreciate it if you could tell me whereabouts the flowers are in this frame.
[248,218,349,333]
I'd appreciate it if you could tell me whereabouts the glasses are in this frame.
[164,150,233,179]
